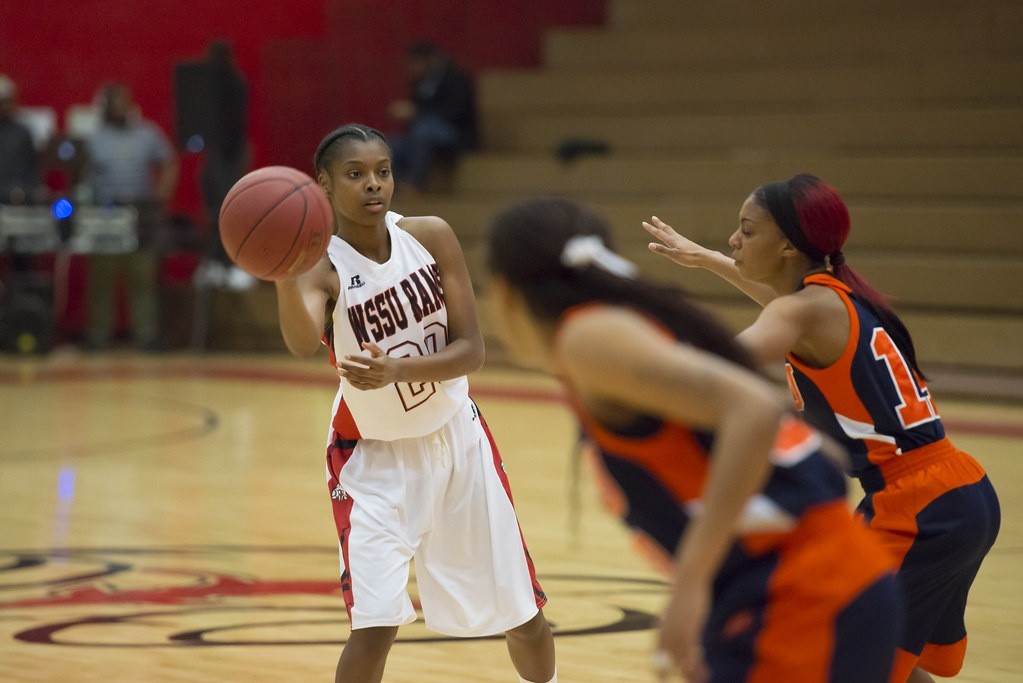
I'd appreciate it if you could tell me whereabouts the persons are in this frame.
[275,121,557,683]
[386,40,480,191]
[47,78,178,352]
[642,175,1001,682]
[489,197,903,683]
[0,73,56,356]
[178,41,248,268]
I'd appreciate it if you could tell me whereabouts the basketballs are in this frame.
[219,165,335,281]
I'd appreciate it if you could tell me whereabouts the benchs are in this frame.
[218,0,1023,400]
